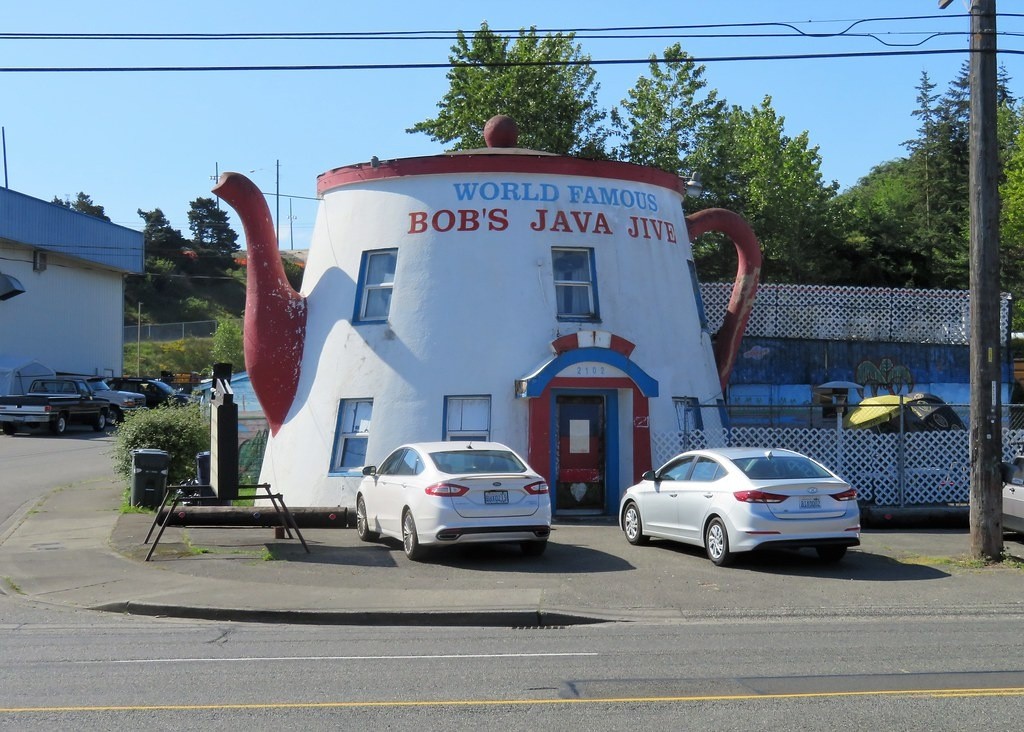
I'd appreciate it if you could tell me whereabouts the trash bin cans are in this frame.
[130,449,170,511]
[196,451,231,506]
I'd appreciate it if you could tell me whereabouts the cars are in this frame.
[355,442,552,561]
[56,374,147,427]
[1001,455,1024,533]
[619,446,861,567]
[105,375,199,409]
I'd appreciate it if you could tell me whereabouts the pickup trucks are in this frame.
[0,376,111,436]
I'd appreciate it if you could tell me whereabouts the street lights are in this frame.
[251,156,280,250]
[137,301,144,377]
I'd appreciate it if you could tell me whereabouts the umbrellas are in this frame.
[842,394,912,432]
[906,392,967,433]
